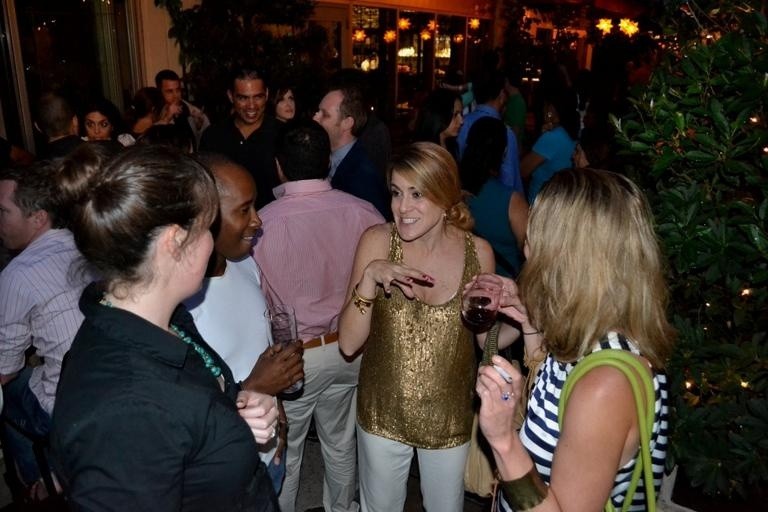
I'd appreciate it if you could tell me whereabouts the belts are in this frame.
[301,332,337,348]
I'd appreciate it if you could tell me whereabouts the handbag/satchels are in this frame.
[464,412,499,498]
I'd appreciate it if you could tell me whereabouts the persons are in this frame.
[0,61,679,512]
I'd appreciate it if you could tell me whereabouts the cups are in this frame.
[264,302,305,400]
[459,270,500,332]
